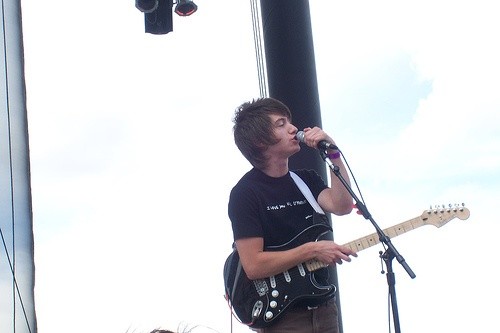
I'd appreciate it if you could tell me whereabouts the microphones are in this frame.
[297,131,339,150]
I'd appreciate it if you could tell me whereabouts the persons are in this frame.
[229,98,357,333]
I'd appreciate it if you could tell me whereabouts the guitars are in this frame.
[223,201,471,331]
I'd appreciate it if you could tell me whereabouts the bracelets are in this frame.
[327,151,340,158]
